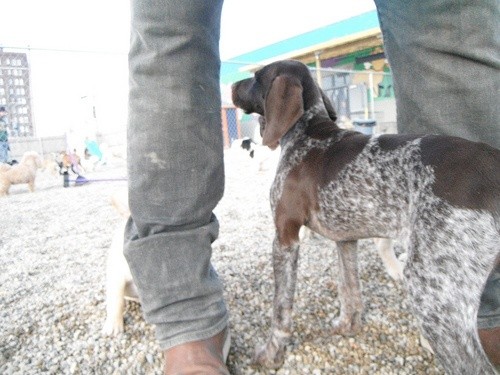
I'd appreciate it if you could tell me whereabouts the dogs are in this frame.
[231,59,500,374]
[0,152,42,197]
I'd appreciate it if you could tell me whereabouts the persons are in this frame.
[128,0,500,375]
[0,105,10,165]
